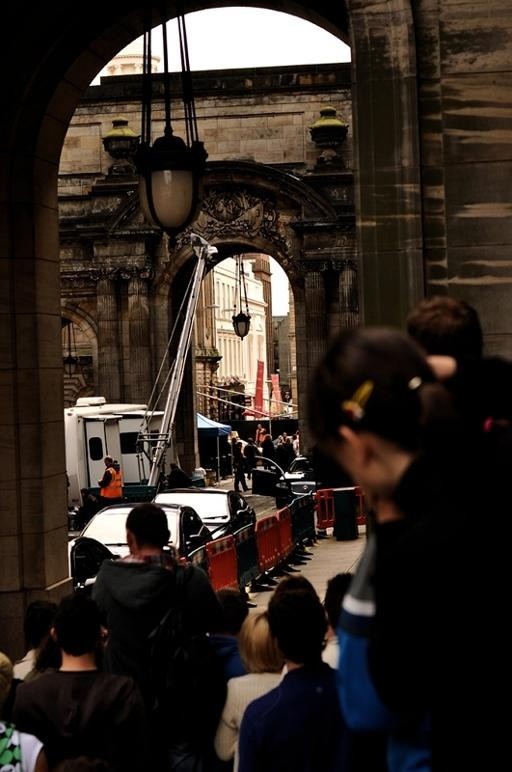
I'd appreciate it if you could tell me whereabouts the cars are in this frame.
[247,450,322,511]
[145,483,260,544]
[66,498,219,589]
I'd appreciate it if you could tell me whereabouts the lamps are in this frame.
[231,253,251,341]
[129,15,209,238]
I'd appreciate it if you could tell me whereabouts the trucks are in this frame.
[64,393,177,509]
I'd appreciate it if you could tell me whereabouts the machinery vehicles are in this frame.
[71,228,224,532]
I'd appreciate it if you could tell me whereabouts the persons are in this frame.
[90,506,223,771]
[98,455,124,505]
[179,588,248,678]
[332,300,490,731]
[24,635,62,683]
[13,600,57,680]
[234,422,300,494]
[2,652,48,769]
[167,462,193,489]
[214,613,288,769]
[238,577,374,770]
[324,573,354,636]
[306,323,510,772]
[473,351,511,420]
[9,593,176,771]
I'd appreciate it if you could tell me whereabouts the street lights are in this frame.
[284,391,290,403]
